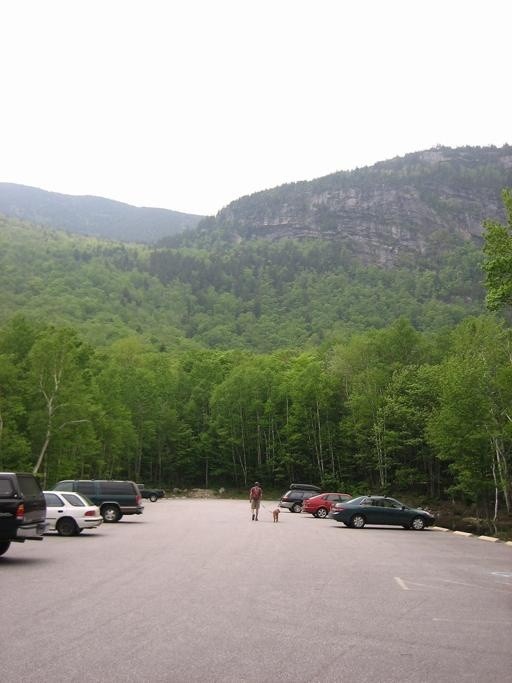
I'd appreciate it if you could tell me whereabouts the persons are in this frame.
[249,482,262,522]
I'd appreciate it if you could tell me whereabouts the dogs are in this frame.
[272,508,281,523]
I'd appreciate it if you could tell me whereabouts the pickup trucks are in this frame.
[136,484,164,501]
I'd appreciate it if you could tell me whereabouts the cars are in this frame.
[328,495,435,530]
[302,492,353,518]
[43,491,103,535]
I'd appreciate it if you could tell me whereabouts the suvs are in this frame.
[278,489,321,512]
[0,472,48,555]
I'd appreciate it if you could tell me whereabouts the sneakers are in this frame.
[252,514,258,520]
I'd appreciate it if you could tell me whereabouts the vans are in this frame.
[54,478,144,522]
